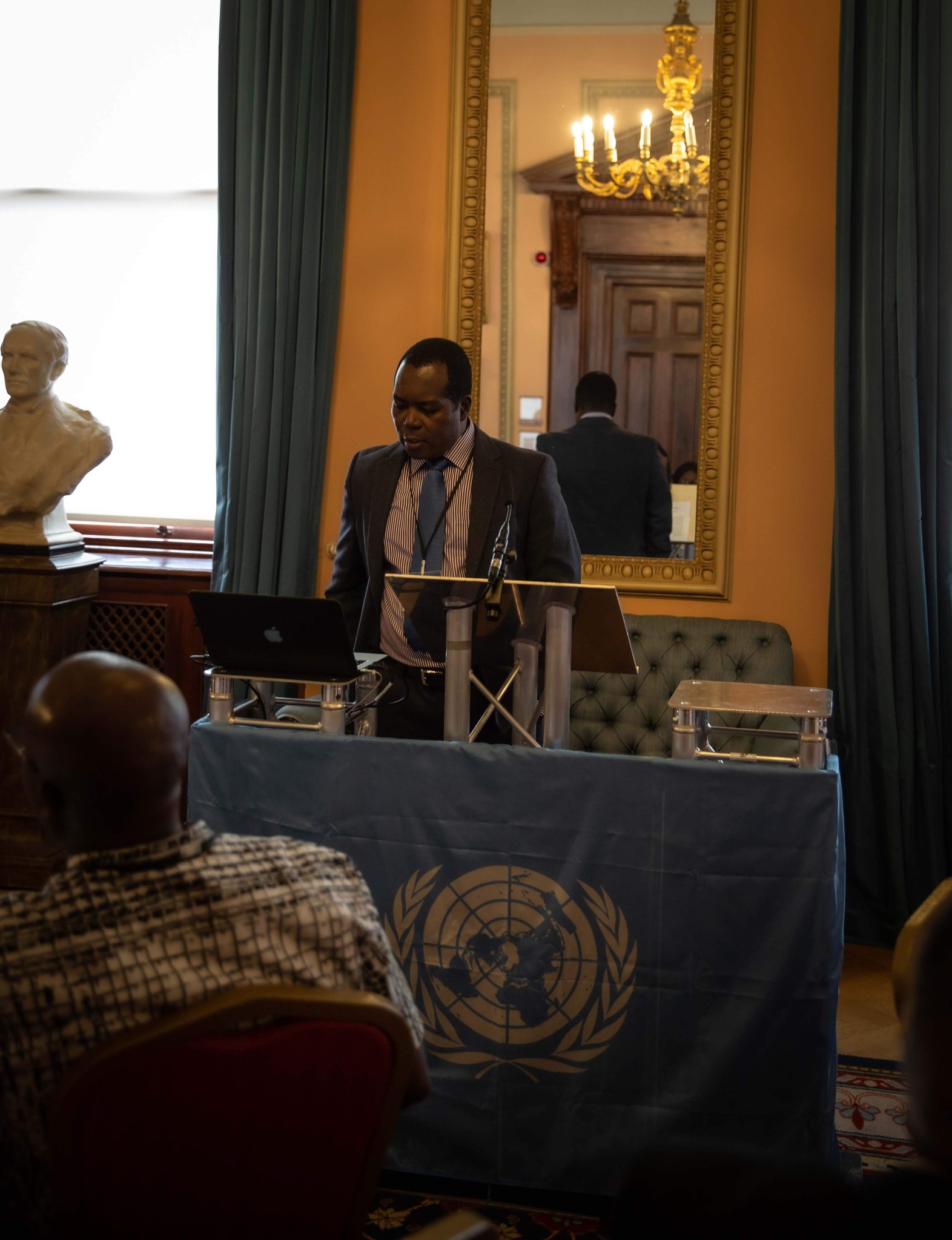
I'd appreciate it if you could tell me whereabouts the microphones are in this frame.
[488,500,515,585]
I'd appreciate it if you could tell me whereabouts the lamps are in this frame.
[573,0,716,220]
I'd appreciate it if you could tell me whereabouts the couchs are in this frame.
[528,618,801,763]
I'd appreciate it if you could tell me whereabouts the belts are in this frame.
[378,648,484,688]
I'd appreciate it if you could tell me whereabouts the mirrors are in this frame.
[438,1,753,599]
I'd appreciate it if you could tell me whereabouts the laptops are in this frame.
[187,590,389,682]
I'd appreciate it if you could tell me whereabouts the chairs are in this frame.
[45,987,417,1240]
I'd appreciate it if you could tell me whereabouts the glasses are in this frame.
[15,748,43,778]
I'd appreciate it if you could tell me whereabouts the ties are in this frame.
[404,457,452,653]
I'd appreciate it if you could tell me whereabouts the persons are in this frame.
[667,460,700,561]
[0,650,430,1240]
[537,371,673,557]
[324,338,586,744]
[0,319,114,551]
